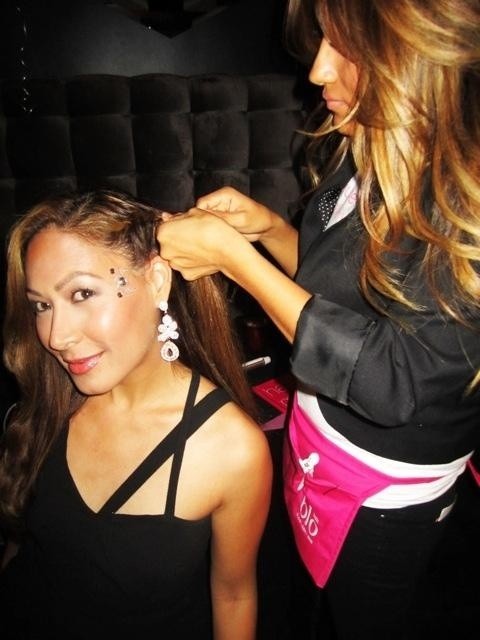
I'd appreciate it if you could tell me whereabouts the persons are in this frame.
[0,190,273,639]
[156,0,480,638]
